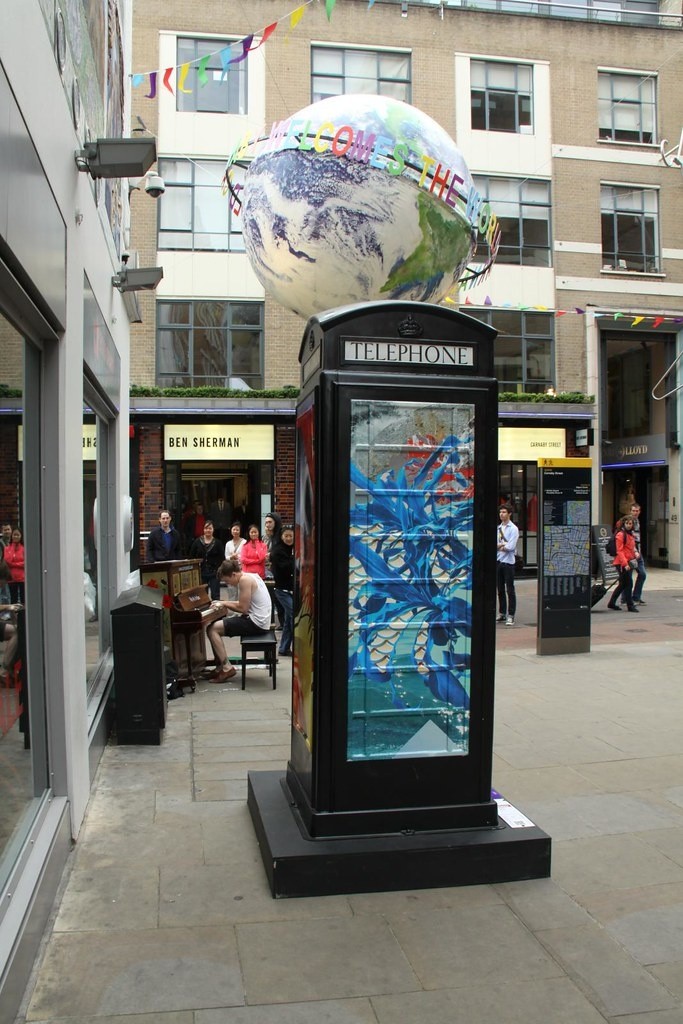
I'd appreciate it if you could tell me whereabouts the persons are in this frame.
[497,503,520,626]
[269,524,295,657]
[0,519,34,752]
[202,559,272,684]
[258,511,285,631]
[188,520,223,601]
[605,515,640,612]
[240,523,268,583]
[224,521,248,617]
[617,503,648,606]
[145,508,183,563]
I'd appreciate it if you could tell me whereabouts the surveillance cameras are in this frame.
[145,176,165,198]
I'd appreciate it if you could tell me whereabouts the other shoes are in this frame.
[608,604,622,611]
[621,599,627,604]
[505,615,515,626]
[278,649,293,656]
[208,667,236,683]
[202,669,222,679]
[633,596,647,604]
[628,606,639,612]
[496,614,506,622]
[276,625,284,630]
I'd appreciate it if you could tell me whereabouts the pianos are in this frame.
[137,557,229,692]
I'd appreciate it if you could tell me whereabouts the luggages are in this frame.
[592,569,631,609]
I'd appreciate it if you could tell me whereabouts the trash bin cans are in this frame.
[108,585,168,746]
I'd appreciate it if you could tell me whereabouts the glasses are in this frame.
[282,523,294,529]
[266,513,272,517]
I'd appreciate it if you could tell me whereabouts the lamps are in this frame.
[75,137,158,179]
[401,0,408,17]
[112,267,165,295]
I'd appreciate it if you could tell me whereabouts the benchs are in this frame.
[240,627,277,690]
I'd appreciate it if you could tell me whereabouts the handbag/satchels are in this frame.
[514,555,524,576]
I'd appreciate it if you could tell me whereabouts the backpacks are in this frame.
[605,528,626,556]
